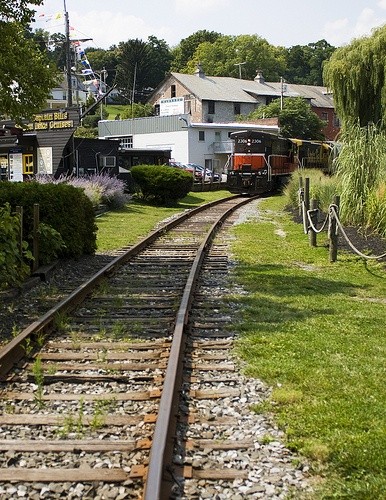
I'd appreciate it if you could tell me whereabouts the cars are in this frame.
[168,158,221,183]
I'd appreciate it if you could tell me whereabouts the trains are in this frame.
[226,130,337,194]
